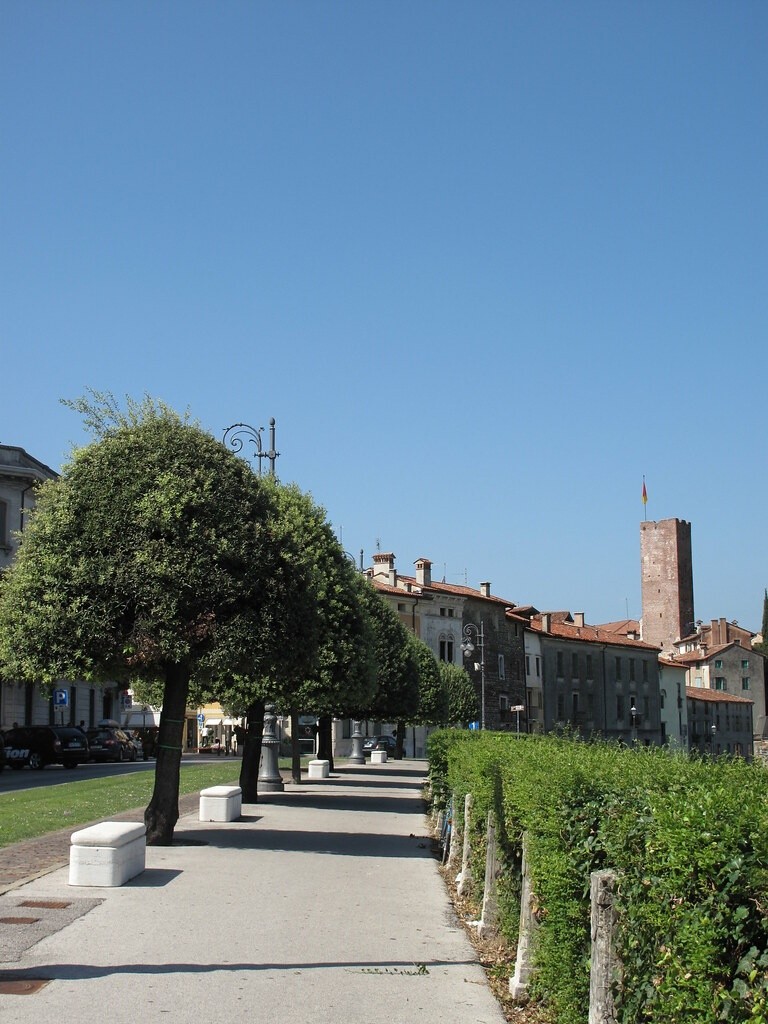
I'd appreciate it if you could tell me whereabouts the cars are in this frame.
[361,735,406,759]
[142,727,159,760]
[123,729,148,762]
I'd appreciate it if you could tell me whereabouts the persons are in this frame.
[201,725,211,748]
[13,722,18,729]
[80,720,88,732]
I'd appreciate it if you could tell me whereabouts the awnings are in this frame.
[205,719,222,725]
[222,718,243,726]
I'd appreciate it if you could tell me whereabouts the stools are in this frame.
[308,759,330,778]
[371,751,387,763]
[69,822,146,887]
[199,786,242,821]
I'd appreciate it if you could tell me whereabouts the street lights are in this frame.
[460,621,485,730]
[711,724,717,762]
[631,706,636,746]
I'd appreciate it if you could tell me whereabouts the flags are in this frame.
[642,484,648,504]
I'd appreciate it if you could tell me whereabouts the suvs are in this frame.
[86,719,138,762]
[3,726,90,769]
